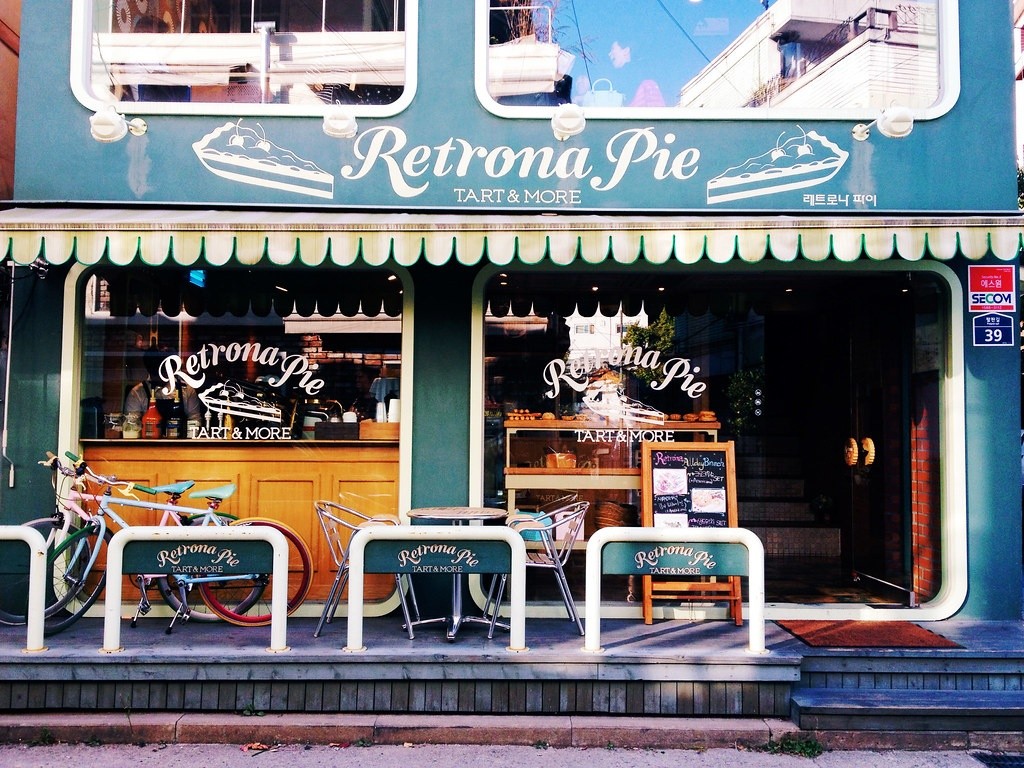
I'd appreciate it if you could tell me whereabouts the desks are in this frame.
[401,507,510,631]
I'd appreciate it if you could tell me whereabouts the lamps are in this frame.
[89,105,147,144]
[552,102,585,142]
[852,101,914,142]
[322,99,358,139]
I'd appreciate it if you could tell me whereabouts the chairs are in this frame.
[313,500,420,640]
[483,501,590,638]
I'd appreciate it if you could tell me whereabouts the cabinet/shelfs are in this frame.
[504,419,721,550]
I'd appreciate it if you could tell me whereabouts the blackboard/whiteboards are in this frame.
[641,440,742,591]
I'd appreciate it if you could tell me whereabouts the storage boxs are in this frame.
[359,422,400,439]
[314,422,359,440]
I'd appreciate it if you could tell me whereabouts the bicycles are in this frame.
[24,451,314,638]
[1,451,272,626]
